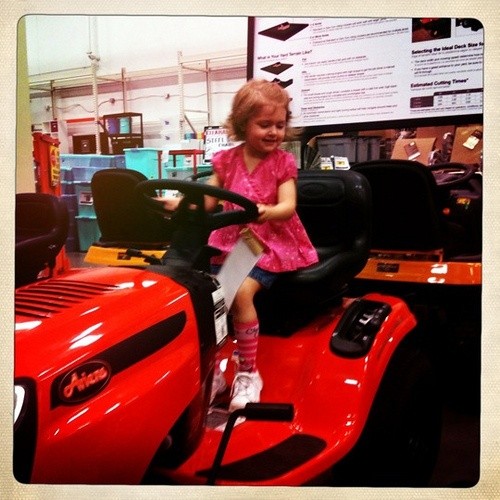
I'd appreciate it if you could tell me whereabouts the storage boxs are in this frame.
[166,165,212,181]
[123,148,184,192]
[60,154,126,251]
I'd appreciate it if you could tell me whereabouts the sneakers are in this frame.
[230,370,263,412]
[210,367,227,405]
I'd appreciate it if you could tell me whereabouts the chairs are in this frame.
[350,160,460,258]
[91,169,168,243]
[15,193,69,288]
[211,170,372,336]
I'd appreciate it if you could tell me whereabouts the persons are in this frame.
[149,80,323,416]
